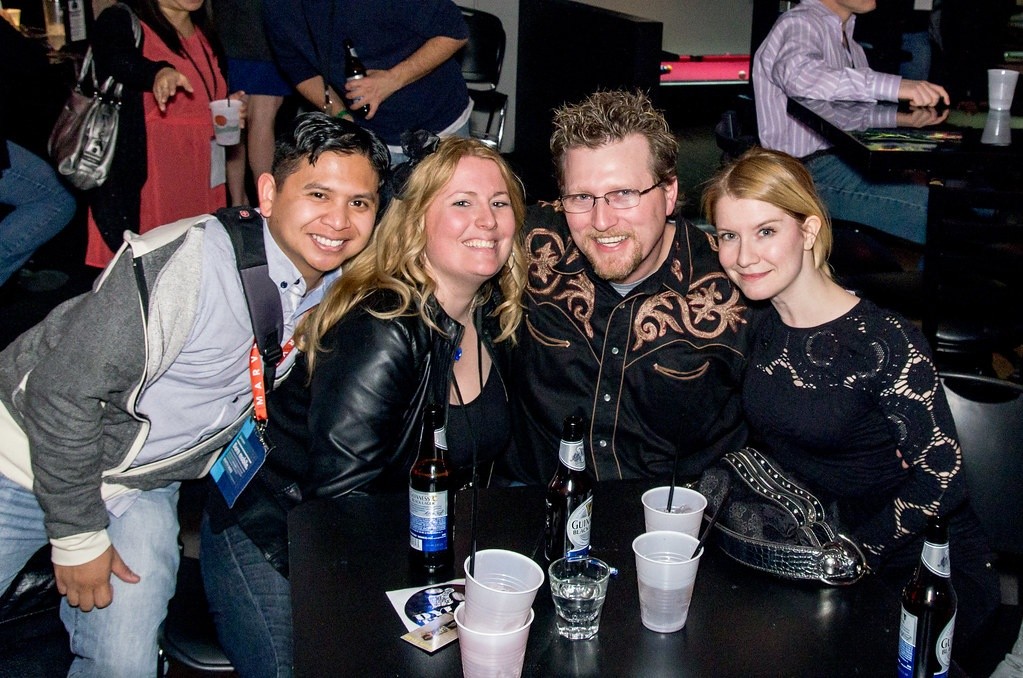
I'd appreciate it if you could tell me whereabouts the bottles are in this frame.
[339,39,370,116]
[544,415,594,563]
[406,402,458,587]
[896,513,957,678]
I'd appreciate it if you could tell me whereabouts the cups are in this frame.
[981,111,1012,146]
[208,99,243,146]
[462,549,545,633]
[641,486,707,539]
[453,600,535,678]
[8,8,21,31]
[632,531,704,634]
[548,555,610,641]
[986,69,1020,112]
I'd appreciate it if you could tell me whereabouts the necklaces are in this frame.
[453,310,470,361]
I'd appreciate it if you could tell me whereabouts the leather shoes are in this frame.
[933,322,1023,354]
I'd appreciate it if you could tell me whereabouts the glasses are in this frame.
[558,179,665,214]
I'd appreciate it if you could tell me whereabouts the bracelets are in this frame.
[336,110,349,118]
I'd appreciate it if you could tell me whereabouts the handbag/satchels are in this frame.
[682,447,866,586]
[48,2,142,190]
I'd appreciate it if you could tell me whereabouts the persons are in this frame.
[796,97,950,132]
[0,14,77,285]
[472,90,774,487]
[261,0,476,170]
[753,0,985,342]
[703,147,1001,677]
[200,135,528,678]
[85,0,248,267]
[224,0,293,219]
[0,111,393,678]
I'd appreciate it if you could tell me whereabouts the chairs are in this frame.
[158,478,238,678]
[454,7,509,147]
[934,372,1023,556]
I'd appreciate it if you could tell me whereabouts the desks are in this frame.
[286,467,972,678]
[788,96,1023,307]
[661,54,750,120]
